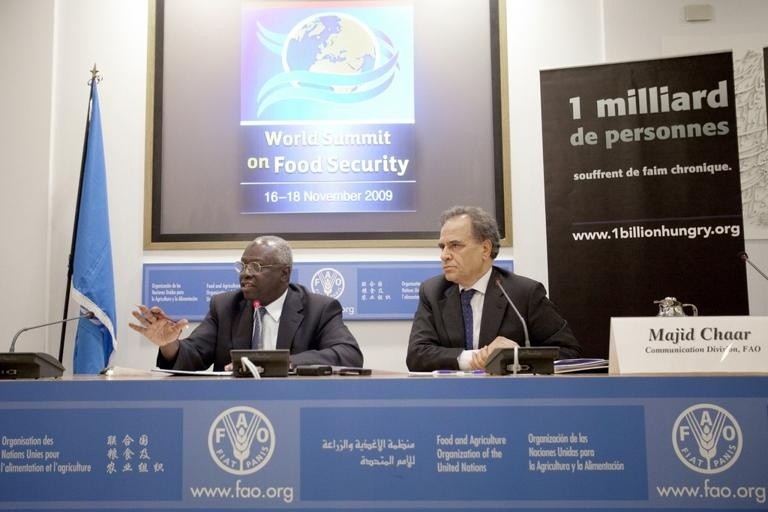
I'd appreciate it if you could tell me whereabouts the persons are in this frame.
[129,236,363,372]
[405,204,584,371]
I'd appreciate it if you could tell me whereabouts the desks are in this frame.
[0,373,768,508]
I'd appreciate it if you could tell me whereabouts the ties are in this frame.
[251,306,266,350]
[461,289,475,350]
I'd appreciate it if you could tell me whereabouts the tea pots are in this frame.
[653,297,698,317]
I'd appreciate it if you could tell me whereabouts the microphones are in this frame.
[0,311,95,379]
[736,252,768,281]
[485,270,560,377]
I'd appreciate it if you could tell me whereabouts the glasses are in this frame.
[234,261,283,274]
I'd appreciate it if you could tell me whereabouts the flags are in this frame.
[71,81,119,375]
